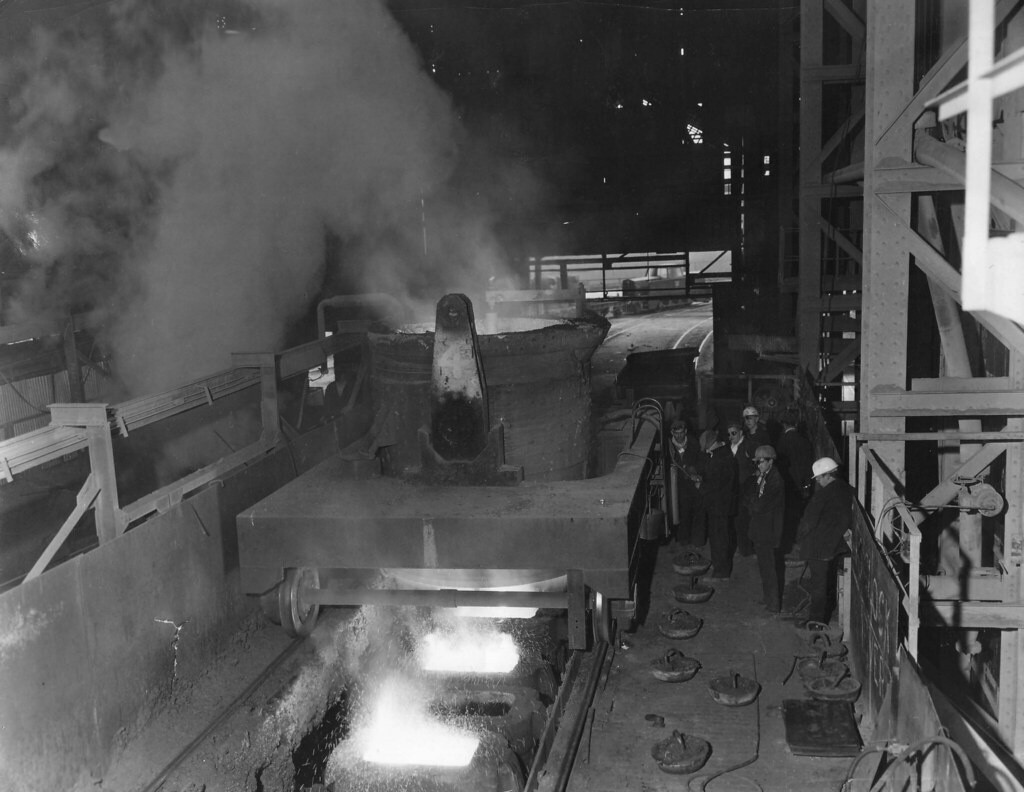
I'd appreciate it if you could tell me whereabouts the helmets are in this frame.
[752,445,777,461]
[743,406,758,418]
[811,457,839,479]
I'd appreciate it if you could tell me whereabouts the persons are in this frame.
[793,456,854,632]
[741,406,771,457]
[666,420,706,547]
[746,445,786,617]
[776,414,813,499]
[722,418,752,558]
[689,428,733,581]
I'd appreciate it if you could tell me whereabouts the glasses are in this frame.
[727,431,739,436]
